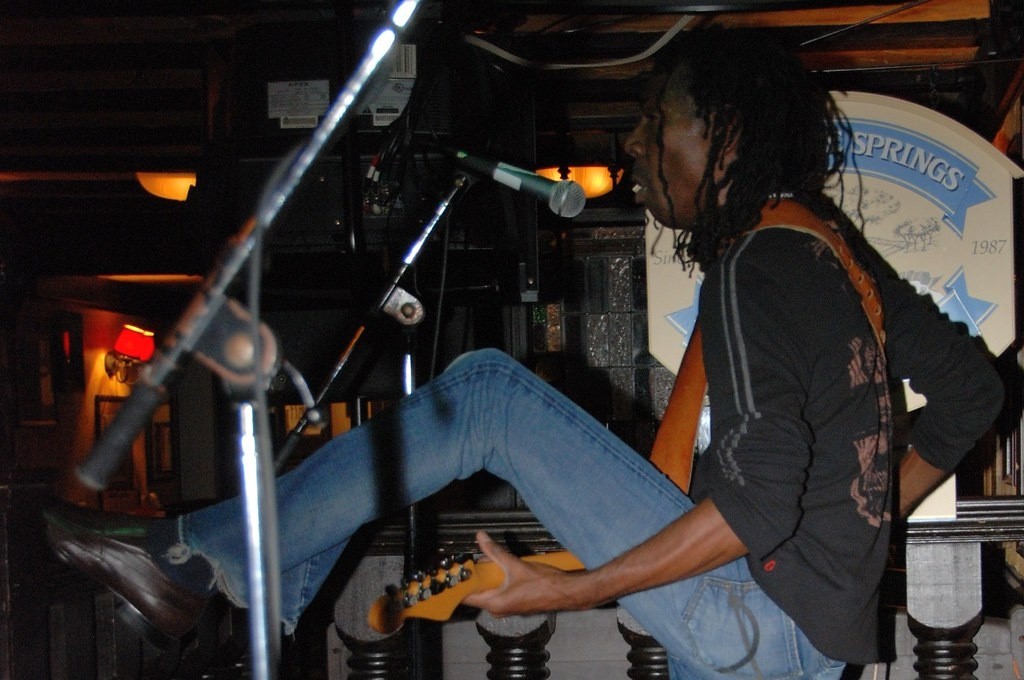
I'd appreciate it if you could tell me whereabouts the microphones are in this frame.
[456,151,586,217]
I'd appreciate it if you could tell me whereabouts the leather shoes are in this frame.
[15,489,213,650]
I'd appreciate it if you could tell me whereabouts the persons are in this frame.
[47,26,1005,680]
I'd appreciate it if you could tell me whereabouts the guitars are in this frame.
[367,552,586,634]
